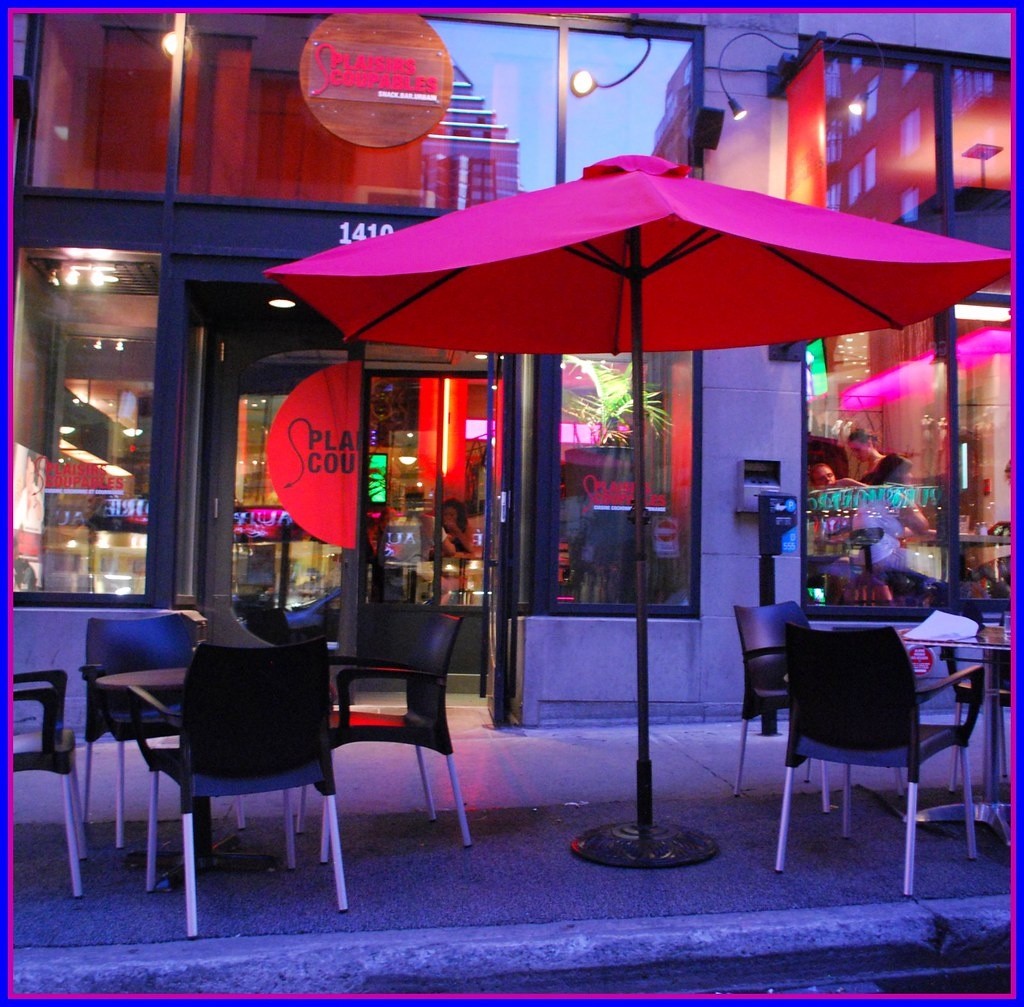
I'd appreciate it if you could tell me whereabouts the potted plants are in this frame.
[562,355,676,575]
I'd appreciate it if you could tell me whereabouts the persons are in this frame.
[12,449,44,591]
[409,498,483,605]
[807,428,950,608]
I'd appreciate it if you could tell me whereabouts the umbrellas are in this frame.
[263,155,1014,867]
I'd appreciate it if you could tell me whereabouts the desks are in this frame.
[94,668,280,894]
[832,624,1011,849]
[902,533,1010,582]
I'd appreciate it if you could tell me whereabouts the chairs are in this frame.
[849,526,927,606]
[940,614,1011,793]
[773,620,987,899]
[144,634,349,940]
[13,669,89,901]
[85,613,246,851]
[732,603,904,814]
[295,609,473,865]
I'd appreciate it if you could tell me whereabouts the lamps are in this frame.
[718,33,804,121]
[825,32,884,116]
[114,14,192,61]
[570,36,651,99]
